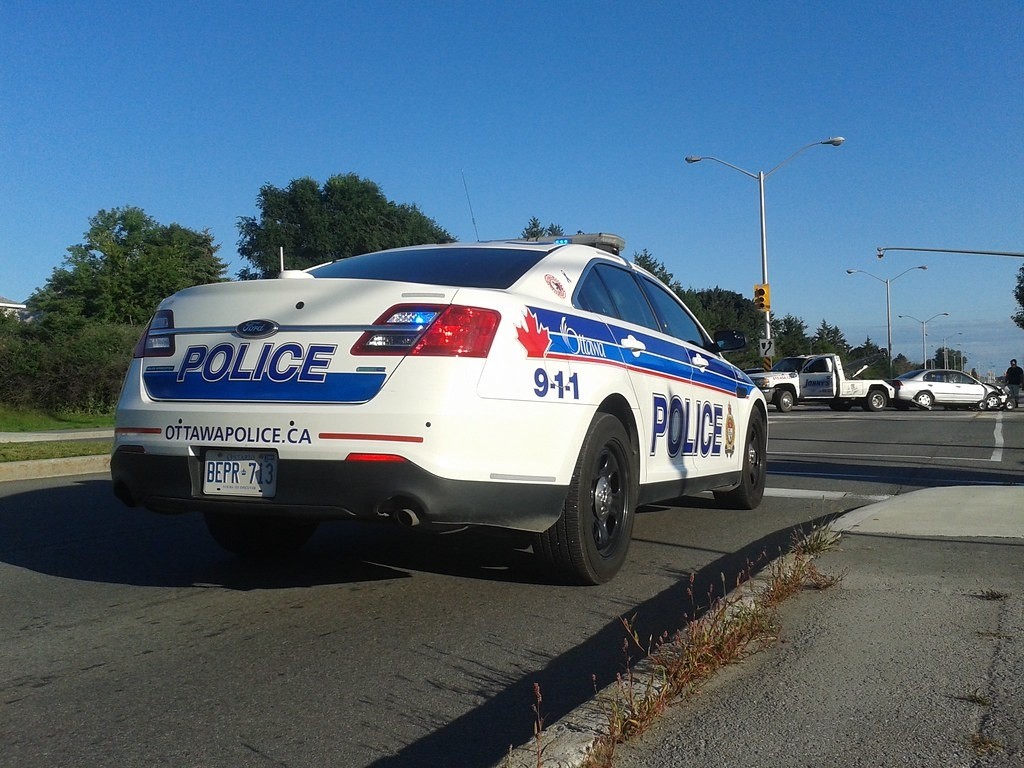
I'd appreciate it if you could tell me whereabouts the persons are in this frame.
[1004,359,1024,408]
[972,368,979,384]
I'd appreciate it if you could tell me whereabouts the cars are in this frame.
[110,232,769,586]
[883,367,1006,411]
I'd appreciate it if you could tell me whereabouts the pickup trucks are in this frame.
[742,351,896,413]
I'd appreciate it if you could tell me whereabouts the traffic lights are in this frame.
[753,282,771,311]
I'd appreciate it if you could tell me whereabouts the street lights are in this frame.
[685,136,845,366]
[846,264,928,378]
[898,312,950,369]
[925,331,962,369]
